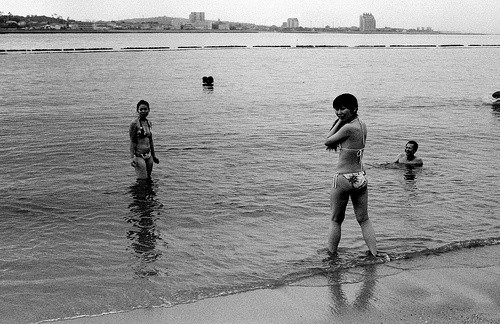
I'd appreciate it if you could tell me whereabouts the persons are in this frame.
[202,76,214,86]
[129,100,160,179]
[323,94,378,257]
[485,90,500,107]
[379,140,423,167]
